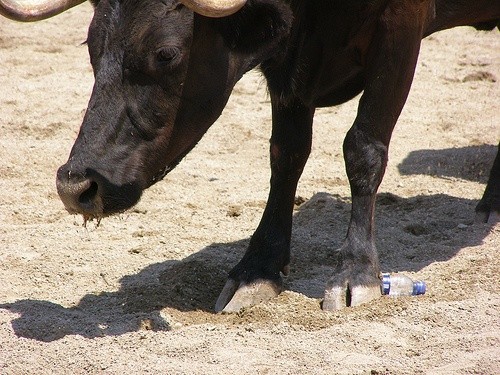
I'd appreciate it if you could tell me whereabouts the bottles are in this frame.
[382,272,425,298]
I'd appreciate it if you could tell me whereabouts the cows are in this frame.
[1,0,500,316]
